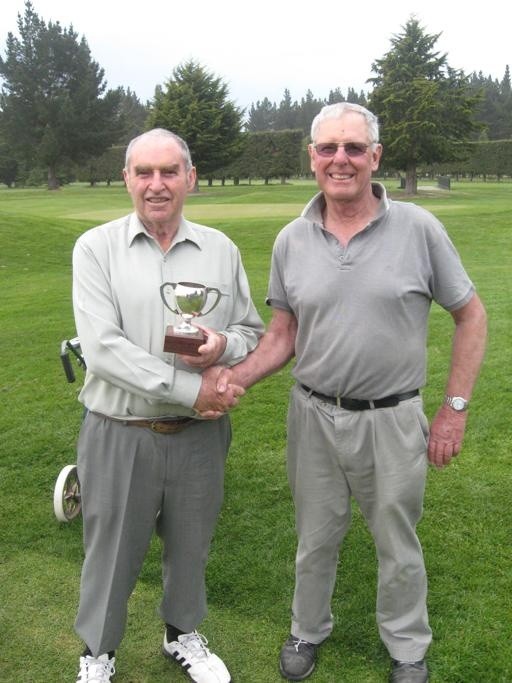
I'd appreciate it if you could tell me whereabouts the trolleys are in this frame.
[53,335,85,523]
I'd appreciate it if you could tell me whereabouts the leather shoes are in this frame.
[390,658,425,683]
[280,634,317,679]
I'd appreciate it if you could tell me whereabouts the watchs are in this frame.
[445,394,468,412]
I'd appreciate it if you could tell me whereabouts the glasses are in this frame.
[312,141,371,157]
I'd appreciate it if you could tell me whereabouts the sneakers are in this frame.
[75,646,115,682]
[163,628,231,683]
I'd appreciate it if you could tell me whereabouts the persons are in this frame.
[200,102,487,683]
[73,128,265,683]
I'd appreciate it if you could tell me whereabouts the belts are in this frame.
[91,410,199,434]
[299,383,419,410]
